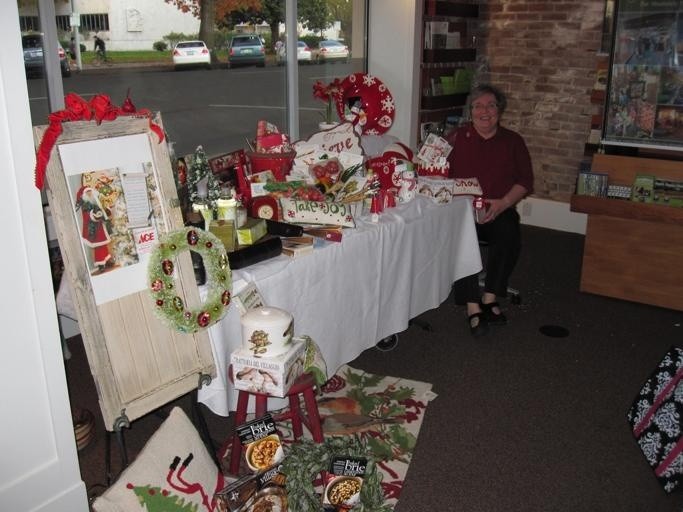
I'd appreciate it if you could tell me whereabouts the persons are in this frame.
[68,37,76,70]
[91,36,107,63]
[437,85,534,337]
[269,39,282,55]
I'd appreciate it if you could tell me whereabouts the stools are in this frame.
[224,374,324,475]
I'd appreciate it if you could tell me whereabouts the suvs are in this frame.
[22,30,71,78]
[226,34,266,69]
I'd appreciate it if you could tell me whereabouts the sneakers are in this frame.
[480,297,507,322]
[468,313,488,337]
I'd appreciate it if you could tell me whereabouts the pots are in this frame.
[240,305,294,358]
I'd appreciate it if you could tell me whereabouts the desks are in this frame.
[139,193,473,418]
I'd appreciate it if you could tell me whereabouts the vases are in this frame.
[71,407,96,451]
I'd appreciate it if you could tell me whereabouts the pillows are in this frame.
[91,405,240,512]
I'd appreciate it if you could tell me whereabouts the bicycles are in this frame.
[90,50,112,67]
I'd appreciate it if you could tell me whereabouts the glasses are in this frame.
[473,103,499,109]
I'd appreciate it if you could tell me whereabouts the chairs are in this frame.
[476,235,522,304]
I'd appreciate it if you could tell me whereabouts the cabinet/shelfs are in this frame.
[569,152,683,312]
[366,0,478,158]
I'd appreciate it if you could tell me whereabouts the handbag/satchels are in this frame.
[628,348,683,494]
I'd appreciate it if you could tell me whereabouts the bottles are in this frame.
[191,182,240,239]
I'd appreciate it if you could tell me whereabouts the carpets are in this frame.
[219,364,432,512]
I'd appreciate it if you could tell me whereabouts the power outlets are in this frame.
[522,202,532,216]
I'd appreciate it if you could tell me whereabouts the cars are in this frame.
[309,40,350,64]
[276,42,312,65]
[172,40,212,70]
[59,41,86,59]
